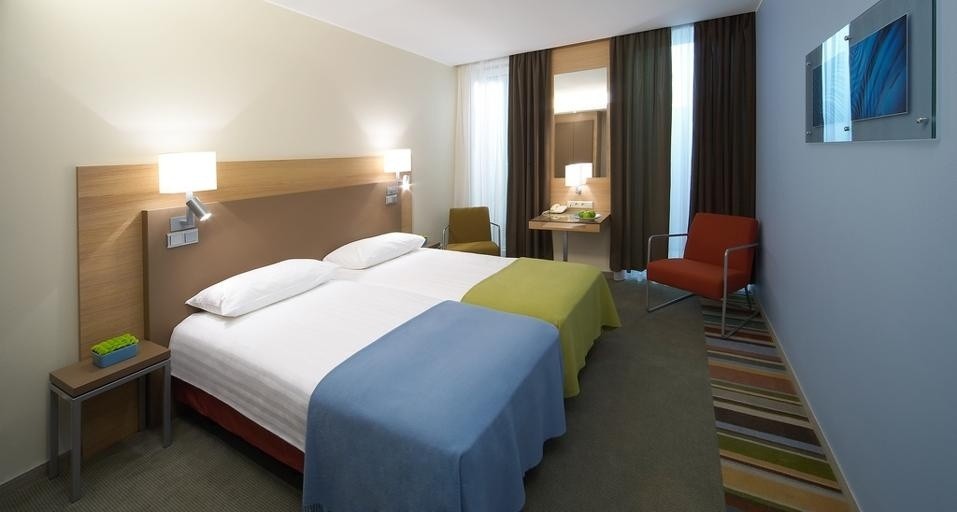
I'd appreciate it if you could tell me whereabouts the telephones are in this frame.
[549,204,567,213]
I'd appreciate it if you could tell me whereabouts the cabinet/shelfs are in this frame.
[554,111,601,178]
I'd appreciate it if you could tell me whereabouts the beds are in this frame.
[169,279,566,512]
[324,248,622,398]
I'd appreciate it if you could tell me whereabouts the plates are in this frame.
[574,213,602,222]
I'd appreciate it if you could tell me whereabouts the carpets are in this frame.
[0,274,726,512]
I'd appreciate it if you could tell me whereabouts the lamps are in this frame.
[565,163,592,194]
[169,197,210,234]
[386,175,410,196]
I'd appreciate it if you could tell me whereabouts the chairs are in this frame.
[442,206,500,256]
[645,213,760,338]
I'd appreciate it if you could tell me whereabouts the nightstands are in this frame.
[47,337,171,503]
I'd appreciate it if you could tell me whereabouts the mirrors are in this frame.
[554,67,606,178]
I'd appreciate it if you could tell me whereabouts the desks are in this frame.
[528,209,611,261]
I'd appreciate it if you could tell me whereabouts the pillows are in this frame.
[185,258,336,318]
[322,232,425,270]
